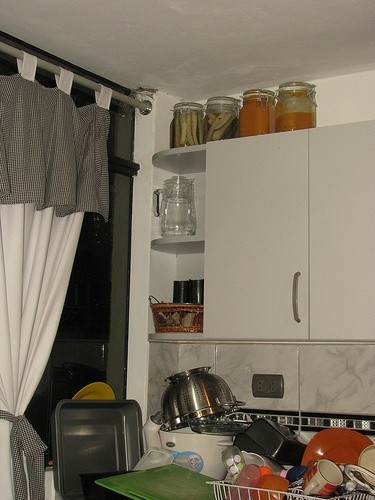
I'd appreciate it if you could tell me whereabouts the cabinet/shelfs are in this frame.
[147,119,375,343]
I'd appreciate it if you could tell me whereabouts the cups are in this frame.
[300,459,344,499]
[173,279,204,305]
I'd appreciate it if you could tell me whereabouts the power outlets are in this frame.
[252,374,285,398]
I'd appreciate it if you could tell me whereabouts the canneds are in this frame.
[169,82,316,150]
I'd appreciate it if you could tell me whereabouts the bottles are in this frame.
[169,81,318,150]
[225,464,261,500]
[222,446,245,480]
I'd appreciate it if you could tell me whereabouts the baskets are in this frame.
[149,295,204,333]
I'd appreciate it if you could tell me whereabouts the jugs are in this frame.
[152,176,197,236]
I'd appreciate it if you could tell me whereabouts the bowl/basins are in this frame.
[237,417,375,500]
[159,427,236,481]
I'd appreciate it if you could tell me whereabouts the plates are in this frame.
[72,382,116,400]
